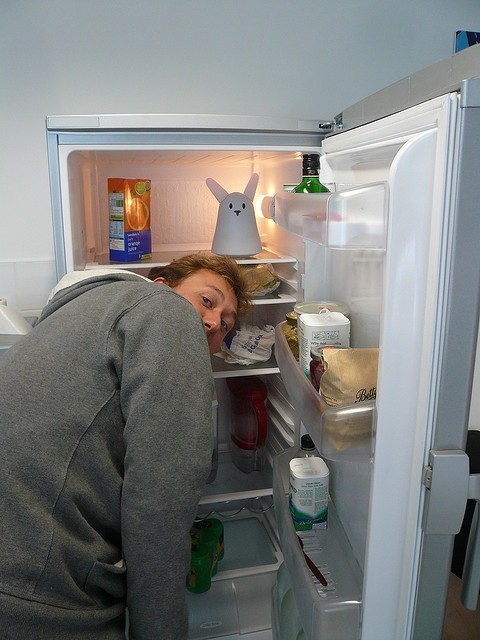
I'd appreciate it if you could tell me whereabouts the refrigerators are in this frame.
[43,44,477,639]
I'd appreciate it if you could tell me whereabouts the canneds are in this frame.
[203,519,225,560]
[309,345,351,396]
[284,312,299,360]
[199,535,218,577]
[186,551,211,594]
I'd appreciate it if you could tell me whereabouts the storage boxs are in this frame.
[187,563,284,639]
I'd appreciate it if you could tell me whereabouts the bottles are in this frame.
[293,154,332,194]
[292,434,319,458]
[206,391,218,484]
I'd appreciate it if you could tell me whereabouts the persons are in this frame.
[1,252,247,640]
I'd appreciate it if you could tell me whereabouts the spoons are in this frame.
[296,534,327,586]
